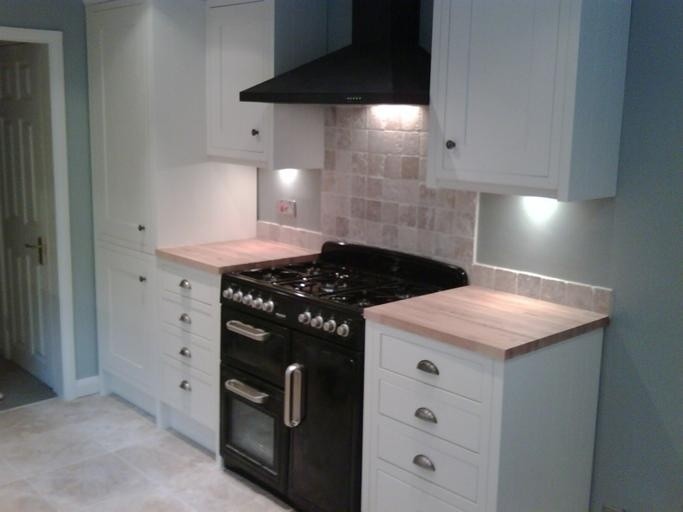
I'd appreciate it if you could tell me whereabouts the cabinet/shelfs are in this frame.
[283,327,363,512]
[160,257,221,460]
[424,1,632,202]
[202,0,328,172]
[359,316,606,512]
[82,0,258,432]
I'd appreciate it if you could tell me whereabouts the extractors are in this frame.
[238,1,432,104]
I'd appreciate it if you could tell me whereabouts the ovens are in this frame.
[221,309,289,495]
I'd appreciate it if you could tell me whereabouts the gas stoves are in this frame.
[220,262,433,336]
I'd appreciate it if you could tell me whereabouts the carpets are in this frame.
[0,357,59,411]
